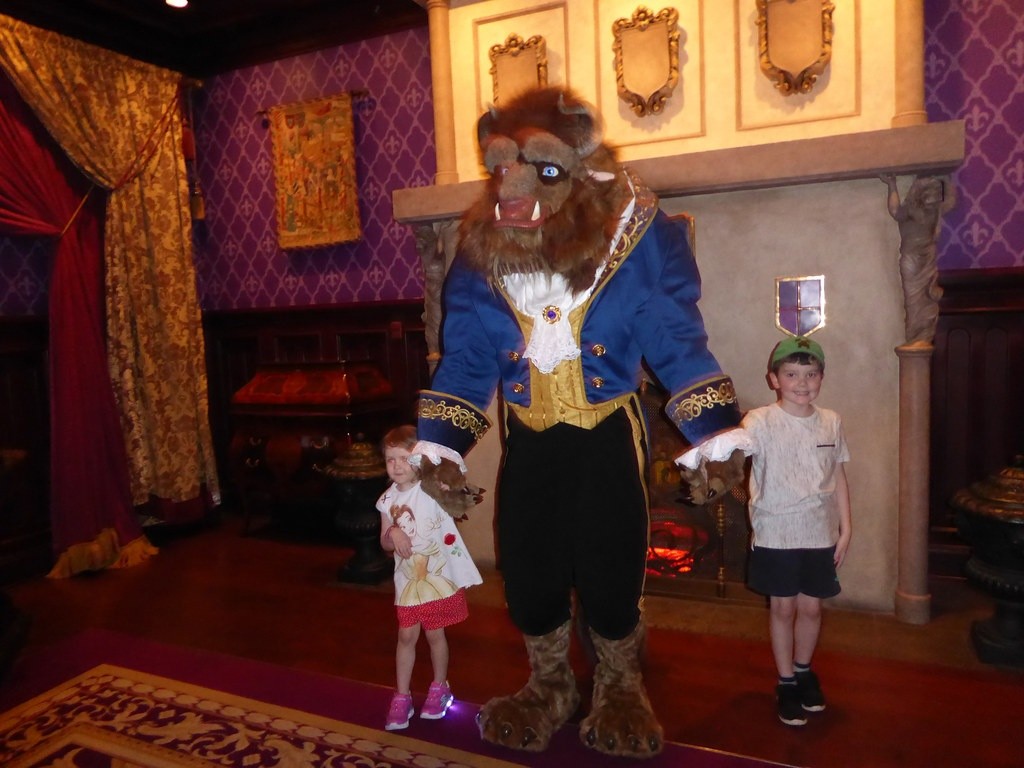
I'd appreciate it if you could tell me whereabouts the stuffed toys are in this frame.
[406,86,753,758]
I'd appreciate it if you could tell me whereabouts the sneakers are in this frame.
[795,670,826,712]
[420,680,454,720]
[385,690,414,731]
[776,683,807,726]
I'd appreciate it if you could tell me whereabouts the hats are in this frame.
[771,336,825,369]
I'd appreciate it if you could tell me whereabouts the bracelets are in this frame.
[384,525,397,544]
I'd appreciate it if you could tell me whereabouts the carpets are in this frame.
[1,663,537,768]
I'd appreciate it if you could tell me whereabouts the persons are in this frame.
[375,427,483,731]
[680,338,851,726]
